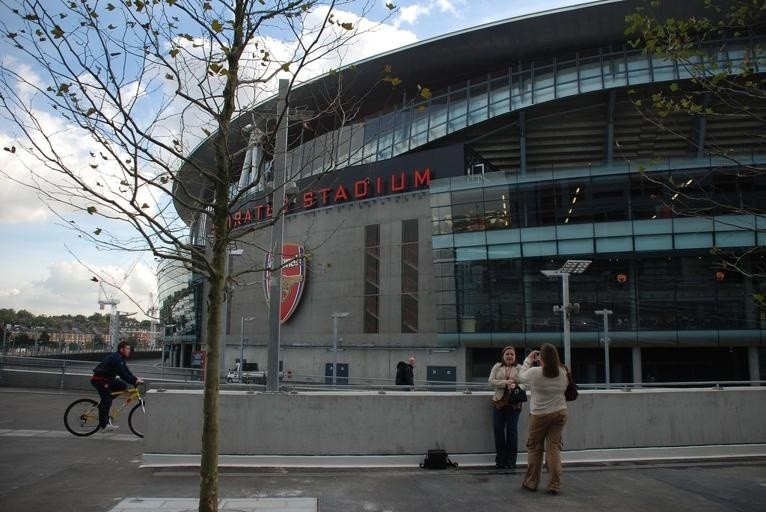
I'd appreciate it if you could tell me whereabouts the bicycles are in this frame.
[64,381,146,438]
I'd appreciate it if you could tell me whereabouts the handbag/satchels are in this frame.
[419,448,459,471]
[564,373,579,401]
[508,390,527,404]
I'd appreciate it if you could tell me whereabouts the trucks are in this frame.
[226,362,283,384]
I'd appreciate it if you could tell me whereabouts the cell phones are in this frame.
[534,351,541,361]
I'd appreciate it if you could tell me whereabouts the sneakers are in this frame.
[544,486,560,496]
[103,418,120,430]
[522,481,537,492]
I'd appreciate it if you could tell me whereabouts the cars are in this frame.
[13,348,28,356]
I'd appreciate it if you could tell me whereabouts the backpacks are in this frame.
[395,361,406,384]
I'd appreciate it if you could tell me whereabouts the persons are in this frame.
[89,340,145,433]
[395,354,416,391]
[486,344,531,469]
[517,342,569,495]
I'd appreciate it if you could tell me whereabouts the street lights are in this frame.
[541,259,593,373]
[219,247,243,375]
[594,310,615,384]
[265,78,300,392]
[238,316,255,384]
[332,312,353,385]
